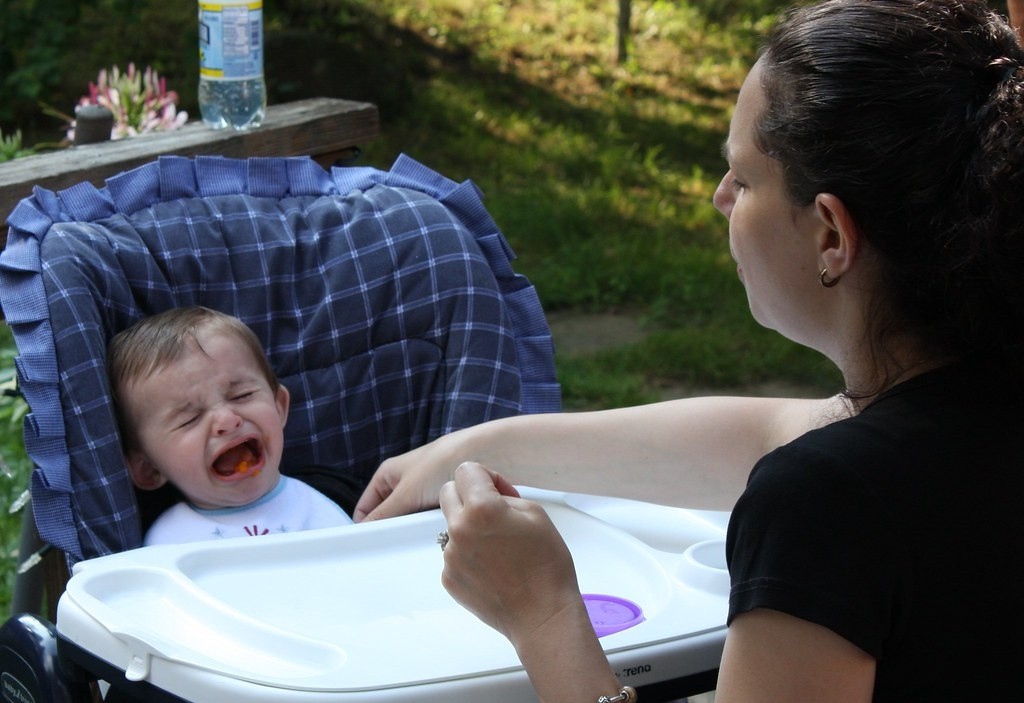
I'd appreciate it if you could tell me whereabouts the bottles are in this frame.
[199,0,267,131]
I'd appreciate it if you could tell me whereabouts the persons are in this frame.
[353,0,1024,703]
[105,304,355,548]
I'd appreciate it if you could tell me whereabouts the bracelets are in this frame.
[596,685,637,703]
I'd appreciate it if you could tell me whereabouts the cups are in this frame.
[683,540,731,594]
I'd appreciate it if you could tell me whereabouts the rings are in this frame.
[436,530,449,552]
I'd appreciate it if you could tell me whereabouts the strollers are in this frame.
[1,153,733,703]
